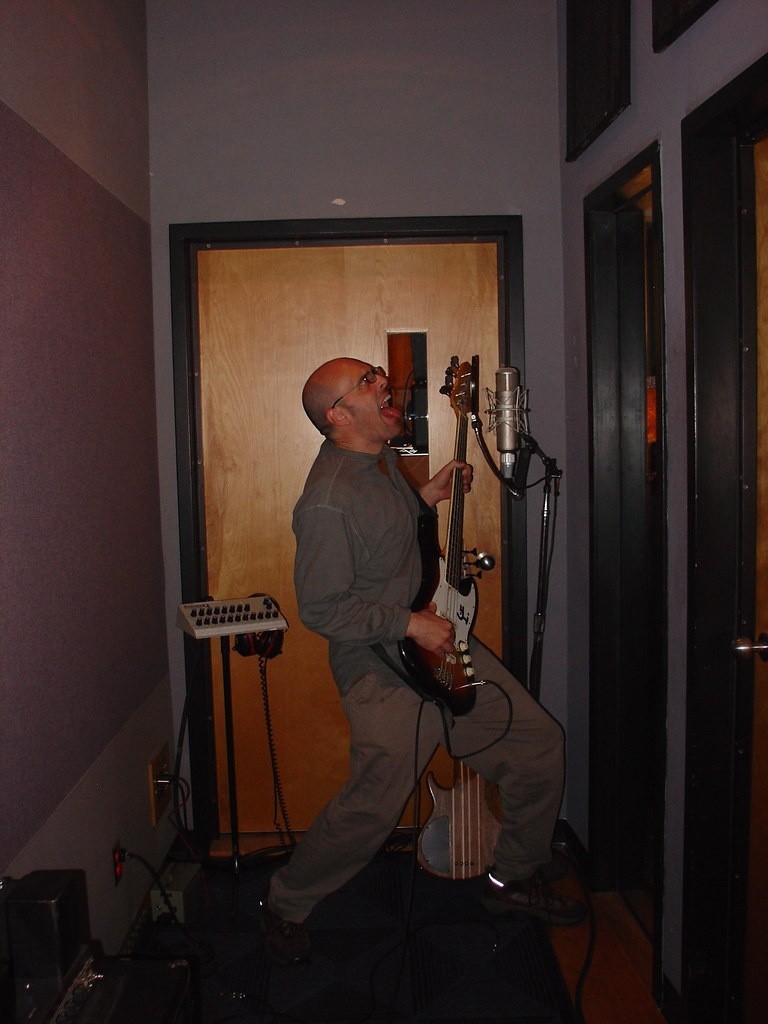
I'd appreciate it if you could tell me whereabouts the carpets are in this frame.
[107,850,580,1024]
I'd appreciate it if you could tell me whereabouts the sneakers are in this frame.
[480,865,587,924]
[257,895,303,971]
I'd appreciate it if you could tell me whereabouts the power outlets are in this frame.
[147,741,172,828]
[112,840,125,888]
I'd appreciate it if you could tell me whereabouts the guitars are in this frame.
[398,355,478,716]
[417,539,503,880]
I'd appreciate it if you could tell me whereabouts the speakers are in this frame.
[5,868,90,1024]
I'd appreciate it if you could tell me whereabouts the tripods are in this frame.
[166,637,299,921]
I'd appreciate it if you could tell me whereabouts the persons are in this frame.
[259,356,588,961]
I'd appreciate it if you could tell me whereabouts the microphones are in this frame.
[495,366,519,485]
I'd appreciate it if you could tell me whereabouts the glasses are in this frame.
[332,366,387,408]
